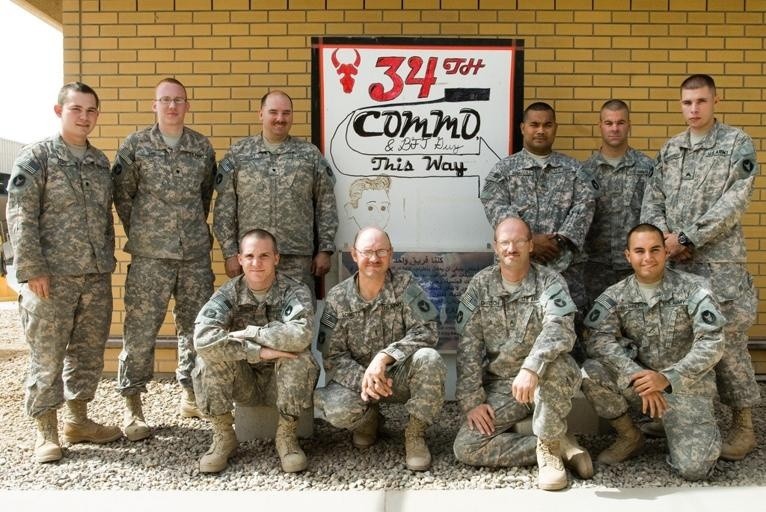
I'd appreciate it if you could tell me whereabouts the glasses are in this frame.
[352,245,394,258]
[153,96,188,106]
[498,238,531,248]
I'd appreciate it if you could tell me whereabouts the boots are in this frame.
[33,409,64,466]
[639,415,669,439]
[594,414,646,468]
[121,393,151,443]
[558,434,594,481]
[352,405,381,451]
[64,399,122,444]
[536,435,569,491]
[404,414,432,473]
[718,408,758,462]
[275,414,308,473]
[198,413,241,475]
[179,387,218,422]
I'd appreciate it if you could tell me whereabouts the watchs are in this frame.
[678,232,691,249]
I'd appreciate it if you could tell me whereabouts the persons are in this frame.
[483,102,596,333]
[580,222,727,479]
[455,216,593,491]
[5,83,122,463]
[192,229,320,474]
[313,226,445,471]
[215,90,340,290]
[642,74,760,460]
[112,79,218,439]
[582,98,653,327]
